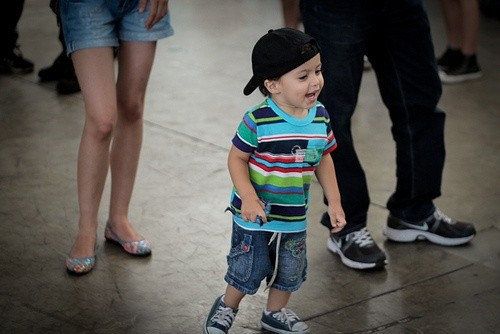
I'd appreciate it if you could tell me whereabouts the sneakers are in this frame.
[39,49,74,83]
[0,52,35,73]
[437,52,483,83]
[382,207,477,247]
[436,47,462,68]
[56,72,81,94]
[203,294,239,334]
[326,224,388,269]
[260,308,311,334]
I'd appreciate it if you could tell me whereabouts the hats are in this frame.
[243,27,322,96]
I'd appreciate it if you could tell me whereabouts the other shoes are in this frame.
[104,221,152,255]
[64,241,96,274]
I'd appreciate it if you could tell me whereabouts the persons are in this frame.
[60,0,175,275]
[300,0,476,270]
[204,28,347,334]
[281,0,483,84]
[0,0,116,96]
